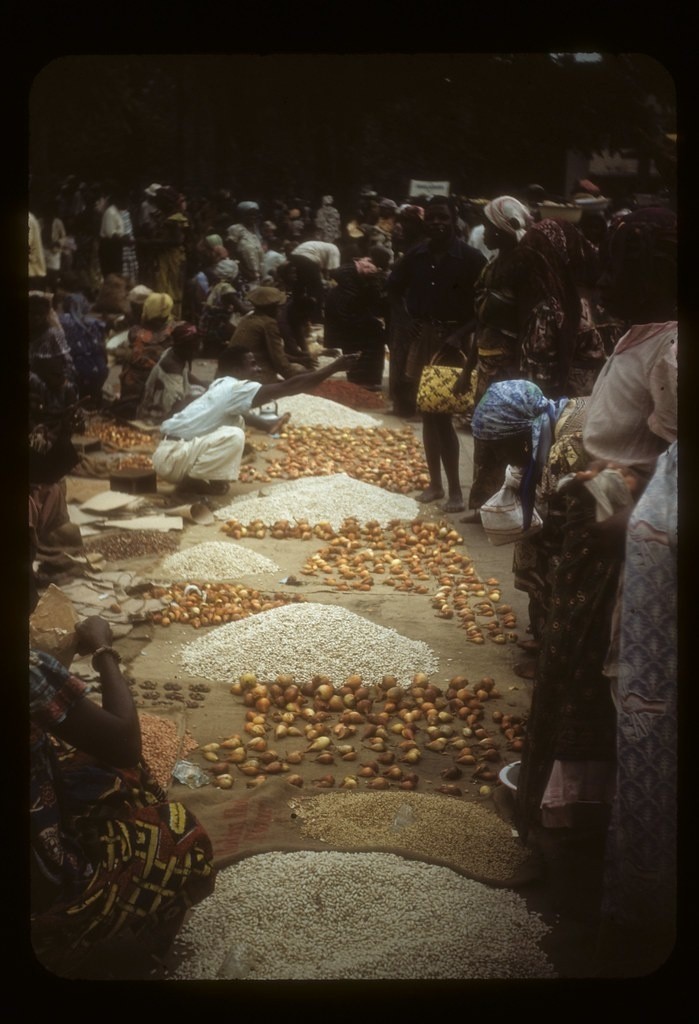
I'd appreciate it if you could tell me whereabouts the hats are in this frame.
[237,201,258,218]
[142,293,173,322]
[205,234,222,250]
[213,259,240,281]
[127,286,155,304]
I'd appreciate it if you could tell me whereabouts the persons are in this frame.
[26,134,678,979]
[153,347,361,495]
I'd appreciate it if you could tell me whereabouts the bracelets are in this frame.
[92,646,121,671]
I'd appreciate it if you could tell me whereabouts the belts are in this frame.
[162,435,186,442]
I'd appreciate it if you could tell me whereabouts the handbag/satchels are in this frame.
[415,349,479,414]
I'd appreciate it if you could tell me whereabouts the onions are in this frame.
[202,673,526,798]
[98,425,156,471]
[237,422,432,495]
[110,517,520,645]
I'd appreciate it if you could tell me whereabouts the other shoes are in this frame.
[37,561,79,580]
[193,480,230,494]
